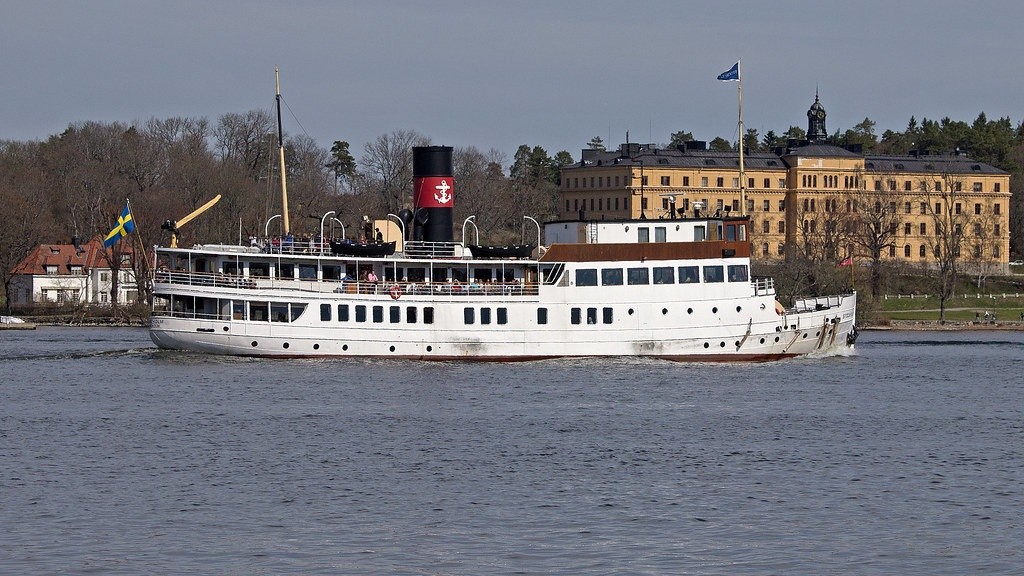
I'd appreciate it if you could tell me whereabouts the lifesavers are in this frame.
[390,286,402,299]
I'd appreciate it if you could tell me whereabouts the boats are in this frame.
[146,65,859,363]
[329,239,397,256]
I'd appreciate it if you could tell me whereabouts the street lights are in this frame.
[993,297,996,318]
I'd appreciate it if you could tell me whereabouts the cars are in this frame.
[1009,260,1024,266]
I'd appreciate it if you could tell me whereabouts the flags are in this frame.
[837,258,852,267]
[104,204,134,248]
[716,60,741,82]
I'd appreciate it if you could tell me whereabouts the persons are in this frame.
[510,279,520,292]
[272,232,326,256]
[417,279,425,290]
[984,311,989,320]
[360,270,378,294]
[976,311,979,320]
[444,278,460,288]
[1020,312,1024,320]
[472,278,499,292]
[375,228,382,244]
